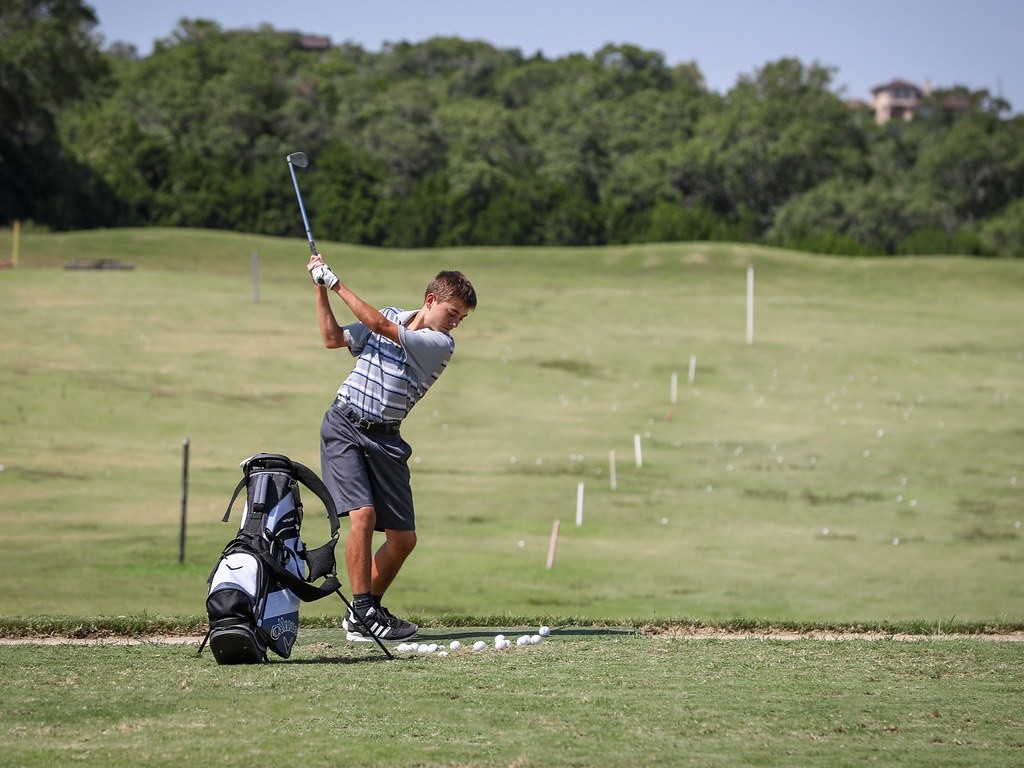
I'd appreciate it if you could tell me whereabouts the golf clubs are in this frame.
[285,150,326,285]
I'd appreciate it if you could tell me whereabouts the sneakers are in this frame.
[343,608,418,643]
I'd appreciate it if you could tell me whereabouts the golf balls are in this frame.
[538,625,551,637]
[395,635,542,658]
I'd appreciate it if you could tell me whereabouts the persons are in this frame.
[305,253,478,645]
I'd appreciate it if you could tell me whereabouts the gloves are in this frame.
[309,259,340,291]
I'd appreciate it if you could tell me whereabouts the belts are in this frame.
[334,398,402,435]
